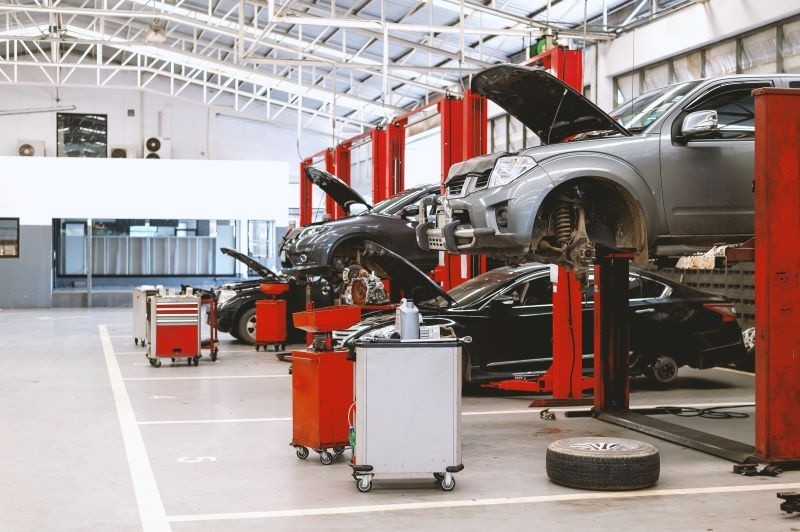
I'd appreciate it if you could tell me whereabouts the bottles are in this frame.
[400,297,421,340]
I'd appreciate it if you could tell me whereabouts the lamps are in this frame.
[144,23,168,44]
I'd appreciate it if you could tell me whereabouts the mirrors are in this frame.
[0,218,20,258]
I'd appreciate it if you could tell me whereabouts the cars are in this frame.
[206,169,443,347]
[415,63,800,266]
[333,240,741,396]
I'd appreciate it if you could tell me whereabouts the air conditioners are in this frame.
[108,144,136,159]
[17,140,46,156]
[144,135,173,159]
